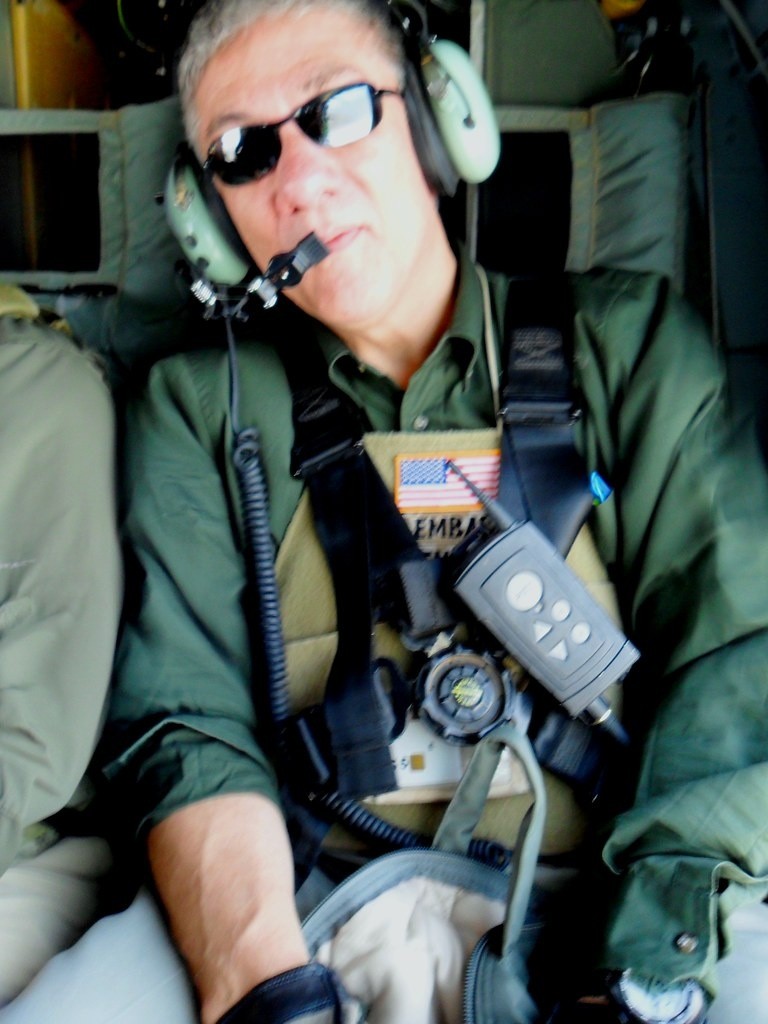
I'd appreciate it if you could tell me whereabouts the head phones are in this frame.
[161,0,501,299]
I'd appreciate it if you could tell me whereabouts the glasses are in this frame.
[203,82,404,186]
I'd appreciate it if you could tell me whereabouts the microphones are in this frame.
[205,232,330,320]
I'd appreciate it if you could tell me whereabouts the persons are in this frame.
[0,277,121,875]
[0,0,768,1024]
